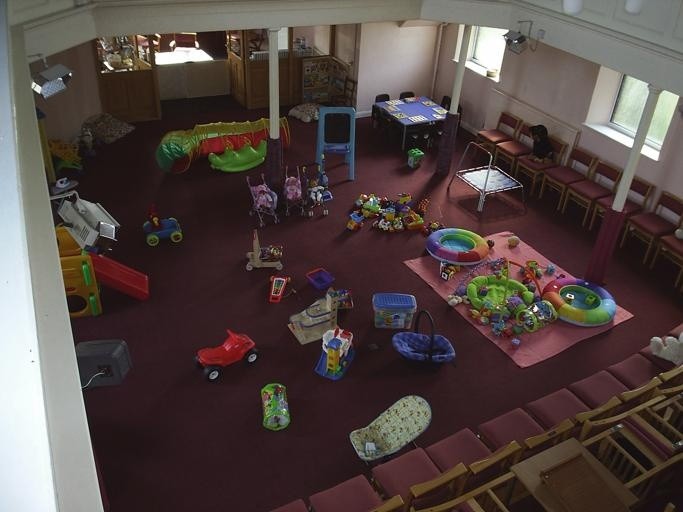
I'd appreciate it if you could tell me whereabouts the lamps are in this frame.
[26,53,74,101]
[502,19,546,55]
[48,189,122,258]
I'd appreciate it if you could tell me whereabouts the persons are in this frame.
[527,125,554,165]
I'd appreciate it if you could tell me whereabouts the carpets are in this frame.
[401,229,636,369]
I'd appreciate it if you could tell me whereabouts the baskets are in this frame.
[372,292,418,330]
[393,309,455,376]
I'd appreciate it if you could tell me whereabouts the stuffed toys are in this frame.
[648,332,682,366]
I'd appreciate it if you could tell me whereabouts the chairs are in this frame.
[369,90,464,149]
[471,111,682,295]
